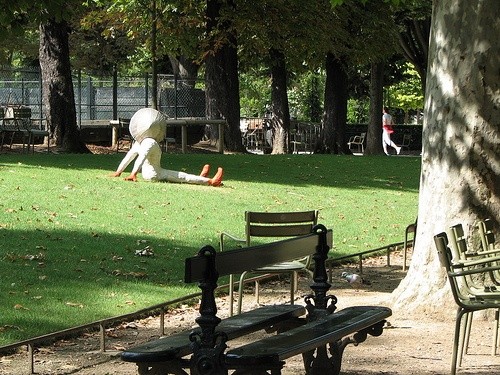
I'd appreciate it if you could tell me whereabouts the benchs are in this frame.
[120,229,392,375]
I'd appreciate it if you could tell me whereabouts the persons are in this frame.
[382,107,402,156]
[106,108,223,187]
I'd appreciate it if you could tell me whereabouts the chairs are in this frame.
[114,115,174,152]
[0,106,50,156]
[433,218,500,375]
[220,209,321,316]
[242,120,411,154]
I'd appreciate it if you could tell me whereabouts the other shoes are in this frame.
[396,146,402,154]
